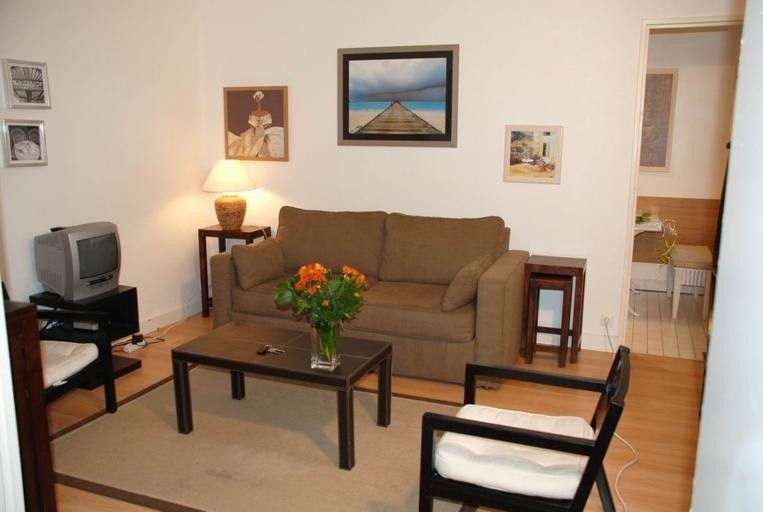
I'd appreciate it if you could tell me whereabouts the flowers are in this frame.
[271,261,368,351]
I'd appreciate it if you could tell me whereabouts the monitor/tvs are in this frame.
[33,222,121,301]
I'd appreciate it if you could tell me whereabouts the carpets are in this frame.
[44,364,476,511]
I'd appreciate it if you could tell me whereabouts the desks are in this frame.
[194,222,269,318]
[519,255,587,368]
[628,214,667,317]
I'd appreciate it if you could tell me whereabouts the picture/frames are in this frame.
[1,118,48,168]
[1,56,52,110]
[337,46,458,148]
[223,86,289,162]
[503,121,563,184]
[640,68,678,174]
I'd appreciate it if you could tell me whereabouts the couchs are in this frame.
[207,206,529,391]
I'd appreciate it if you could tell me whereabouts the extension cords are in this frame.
[123,340,145,353]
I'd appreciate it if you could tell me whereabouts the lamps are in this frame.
[199,161,259,230]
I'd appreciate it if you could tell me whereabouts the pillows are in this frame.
[440,245,507,312]
[228,237,281,291]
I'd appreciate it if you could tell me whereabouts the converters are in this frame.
[132,334,143,345]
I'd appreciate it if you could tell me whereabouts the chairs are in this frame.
[0,280,143,416]
[412,346,632,512]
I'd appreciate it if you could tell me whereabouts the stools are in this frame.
[666,242,713,321]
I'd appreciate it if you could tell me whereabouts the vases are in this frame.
[302,316,340,369]
